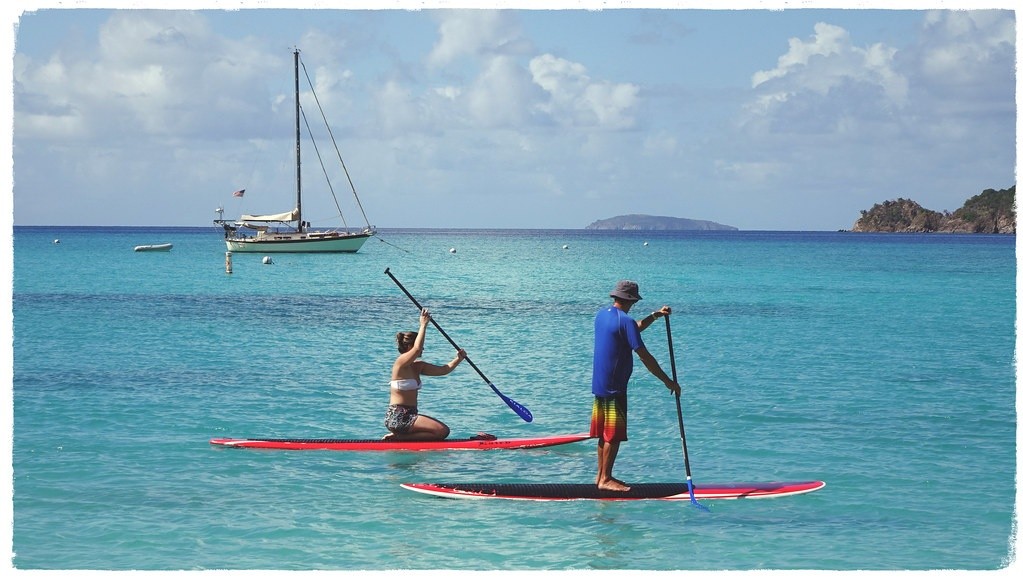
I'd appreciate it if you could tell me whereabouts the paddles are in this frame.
[383,266,535,423]
[662,305,711,517]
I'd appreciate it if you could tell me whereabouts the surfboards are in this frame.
[396,477,827,503]
[207,431,596,452]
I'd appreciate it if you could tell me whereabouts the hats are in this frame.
[610,281,642,301]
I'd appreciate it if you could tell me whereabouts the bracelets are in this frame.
[651,312,659,321]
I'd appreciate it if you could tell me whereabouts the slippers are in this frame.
[469,432,498,440]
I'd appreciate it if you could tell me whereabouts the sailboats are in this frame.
[212,47,378,254]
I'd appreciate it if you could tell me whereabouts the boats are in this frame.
[134,243,173,252]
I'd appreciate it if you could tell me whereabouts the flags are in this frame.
[232,190,245,197]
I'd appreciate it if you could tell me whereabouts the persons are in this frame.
[381,308,465,441]
[590,280,681,497]
